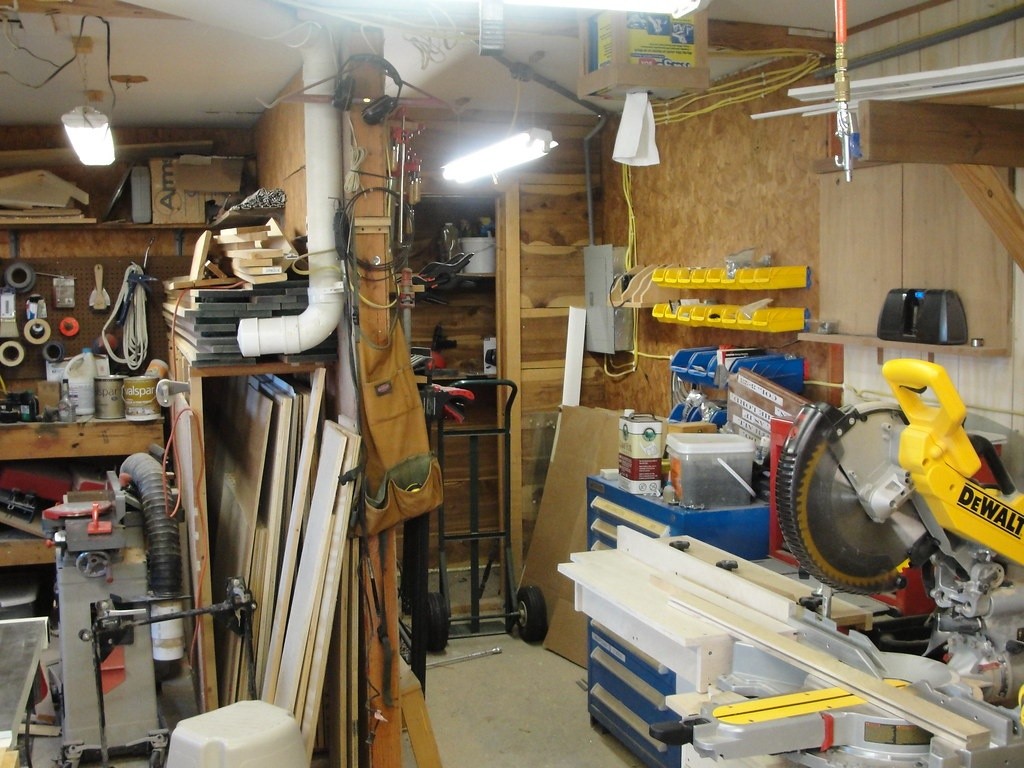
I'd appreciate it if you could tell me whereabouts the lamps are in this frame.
[442,61,560,184]
[61,36,116,166]
[505,0,712,19]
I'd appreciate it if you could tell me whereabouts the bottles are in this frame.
[63,348,95,416]
[59,379,75,422]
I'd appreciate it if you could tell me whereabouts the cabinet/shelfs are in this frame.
[394,272,497,383]
[669,346,805,433]
[652,264,811,334]
[0,223,220,566]
[586,473,771,768]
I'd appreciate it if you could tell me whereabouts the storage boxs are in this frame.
[69,465,105,491]
[0,460,73,502]
[130,166,152,223]
[618,416,663,497]
[150,157,207,223]
[45,354,110,382]
[663,431,756,508]
[668,421,718,435]
[37,379,61,421]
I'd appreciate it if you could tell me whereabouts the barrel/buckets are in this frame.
[619,415,662,497]
[94,375,159,421]
[667,433,754,508]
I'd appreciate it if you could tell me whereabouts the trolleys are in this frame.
[417,372,546,656]
